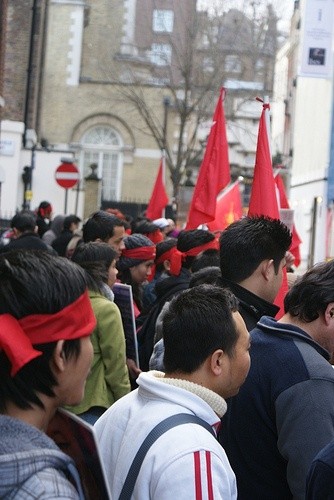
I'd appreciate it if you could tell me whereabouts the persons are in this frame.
[217,257,334,500]
[93,283,251,500]
[0,250,97,500]
[0,201,295,425]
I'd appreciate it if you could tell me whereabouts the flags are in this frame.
[247,97,290,323]
[206,184,243,233]
[145,160,169,220]
[273,174,302,266]
[186,88,231,230]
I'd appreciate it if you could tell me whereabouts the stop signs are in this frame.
[54,163,80,188]
